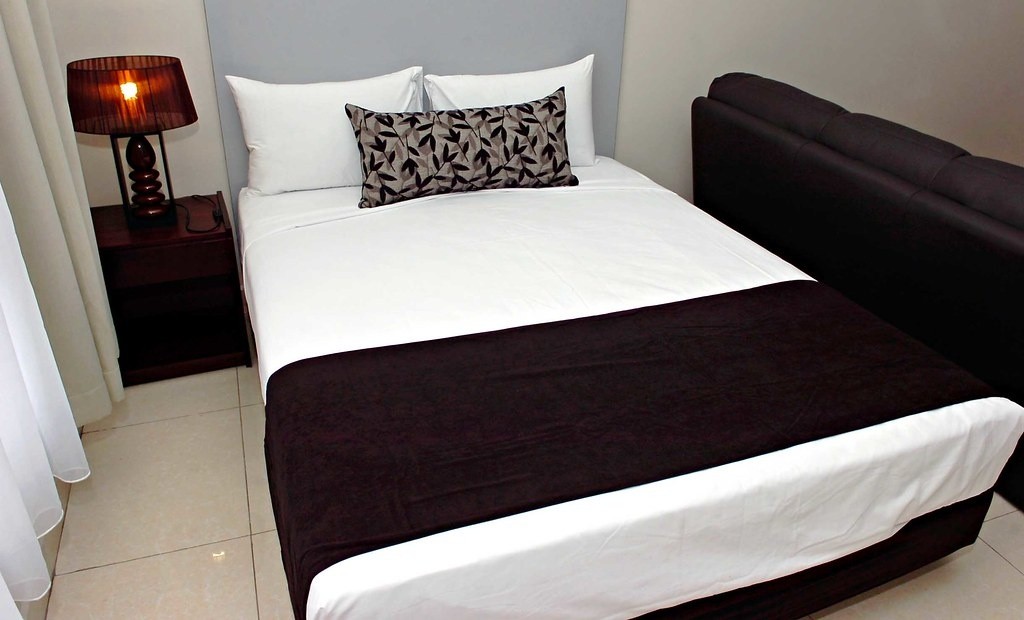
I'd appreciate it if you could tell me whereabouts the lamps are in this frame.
[65,55,201,230]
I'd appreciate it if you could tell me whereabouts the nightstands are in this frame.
[89,191,255,390]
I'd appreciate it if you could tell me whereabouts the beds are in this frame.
[202,0,1024,620]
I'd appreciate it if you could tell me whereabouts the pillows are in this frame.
[426,51,603,166]
[224,65,425,196]
[345,85,579,209]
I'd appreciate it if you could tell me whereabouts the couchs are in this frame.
[691,71,1022,517]
